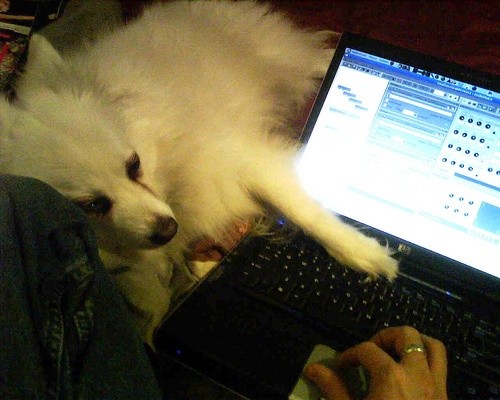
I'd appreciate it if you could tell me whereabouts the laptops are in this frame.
[151,29,500,400]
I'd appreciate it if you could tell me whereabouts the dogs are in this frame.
[0,0,400,296]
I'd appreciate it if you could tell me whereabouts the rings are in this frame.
[399,344,425,356]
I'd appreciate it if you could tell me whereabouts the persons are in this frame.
[0,176,448,400]
